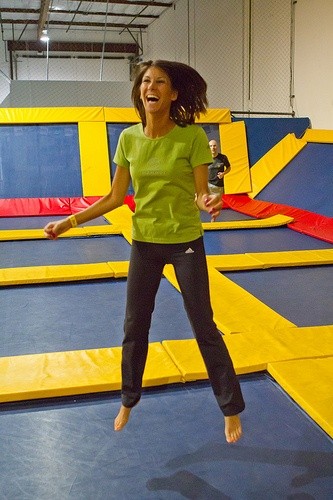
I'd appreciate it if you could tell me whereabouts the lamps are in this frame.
[40,25,50,42]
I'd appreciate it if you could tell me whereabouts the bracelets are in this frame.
[68,215,79,228]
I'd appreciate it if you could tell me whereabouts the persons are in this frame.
[43,59,250,446]
[205,138,232,196]
